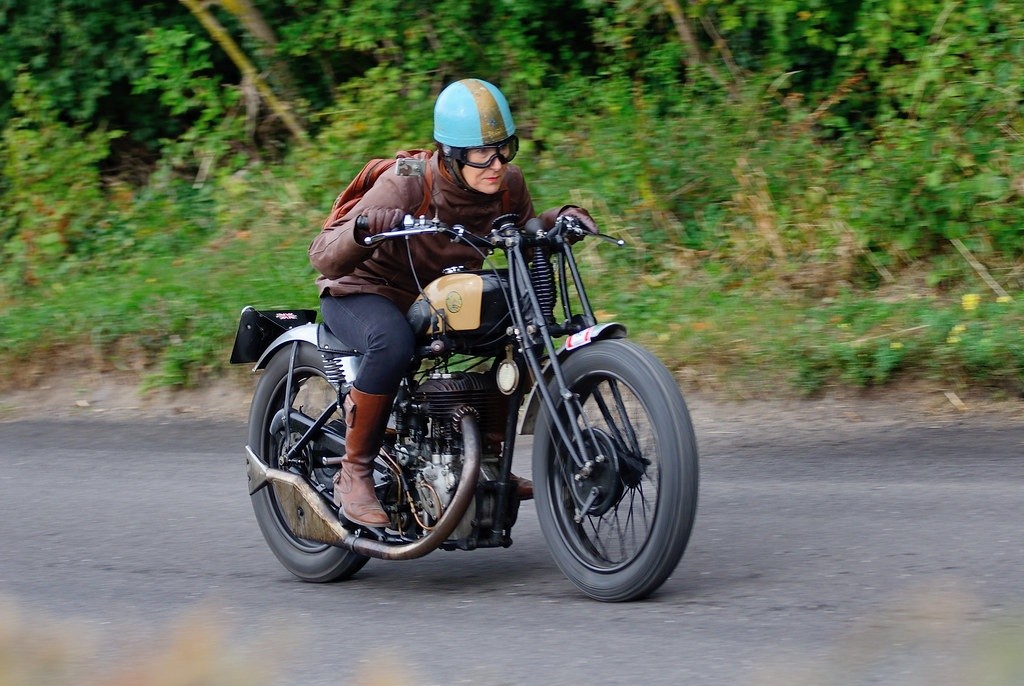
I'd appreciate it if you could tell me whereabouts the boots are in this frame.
[490,433,534,500]
[333,380,394,527]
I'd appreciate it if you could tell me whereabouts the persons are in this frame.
[310,77,599,527]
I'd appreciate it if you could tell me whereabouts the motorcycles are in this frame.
[227,214,702,606]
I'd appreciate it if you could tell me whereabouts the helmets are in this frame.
[433,79,516,147]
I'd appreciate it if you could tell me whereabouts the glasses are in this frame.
[460,135,519,168]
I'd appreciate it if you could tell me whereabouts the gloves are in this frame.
[559,205,599,245]
[360,206,405,236]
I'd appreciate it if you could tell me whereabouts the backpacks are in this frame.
[322,149,509,240]
